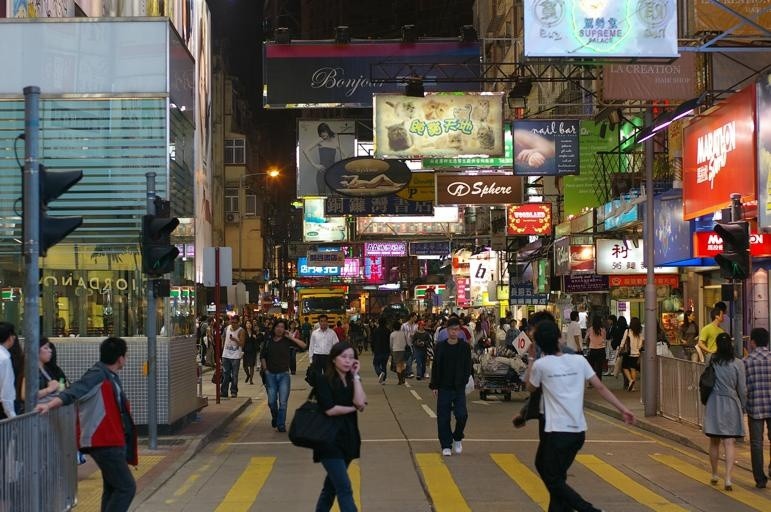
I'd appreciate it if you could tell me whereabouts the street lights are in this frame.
[237,167,280,280]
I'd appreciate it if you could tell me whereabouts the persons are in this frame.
[524,318,638,512]
[335,174,403,190]
[1,300,168,511]
[739,325,771,490]
[312,340,371,511]
[700,331,749,493]
[167,300,733,466]
[304,121,349,195]
[513,129,556,169]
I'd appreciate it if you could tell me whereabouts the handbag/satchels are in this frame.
[290,400,336,449]
[306,367,317,386]
[212,368,231,383]
[619,336,630,356]
[699,366,715,405]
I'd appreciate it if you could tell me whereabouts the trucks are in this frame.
[298,287,348,333]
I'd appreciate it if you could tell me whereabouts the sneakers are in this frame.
[724,480,732,491]
[408,372,430,380]
[246,375,253,384]
[272,417,286,432]
[756,481,766,488]
[625,379,636,392]
[603,371,614,376]
[711,475,718,484]
[378,372,385,385]
[452,438,462,454]
[219,392,237,397]
[442,447,452,456]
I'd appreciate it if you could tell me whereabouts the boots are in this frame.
[397,369,405,384]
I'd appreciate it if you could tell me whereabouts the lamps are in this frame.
[606,183,646,220]
[273,25,477,45]
[405,78,533,109]
[599,88,736,146]
[471,245,488,256]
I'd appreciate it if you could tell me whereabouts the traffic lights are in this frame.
[19,164,84,257]
[716,221,749,282]
[139,214,178,278]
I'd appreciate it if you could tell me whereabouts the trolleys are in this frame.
[475,350,514,401]
